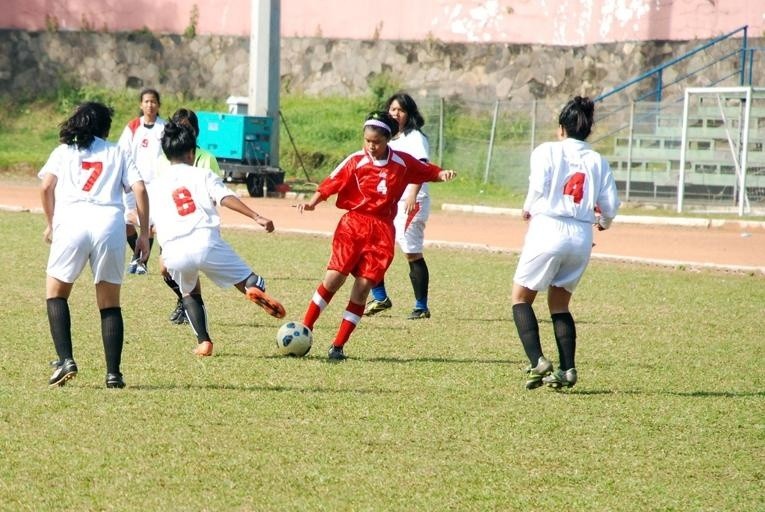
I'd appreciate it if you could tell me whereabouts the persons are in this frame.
[287,109,459,363]
[116,88,174,276]
[31,100,152,389]
[156,107,222,327]
[126,114,286,357]
[510,95,623,391]
[361,90,435,322]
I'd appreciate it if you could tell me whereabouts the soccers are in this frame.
[277,322,313,357]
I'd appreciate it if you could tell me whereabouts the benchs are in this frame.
[599,78,764,193]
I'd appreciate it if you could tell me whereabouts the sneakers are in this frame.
[106,372,126,389]
[170,298,189,325]
[405,308,431,320]
[125,256,148,274]
[362,296,394,318]
[524,355,553,389]
[328,345,347,360]
[245,286,287,319]
[47,356,79,387]
[542,367,578,389]
[191,342,214,356]
